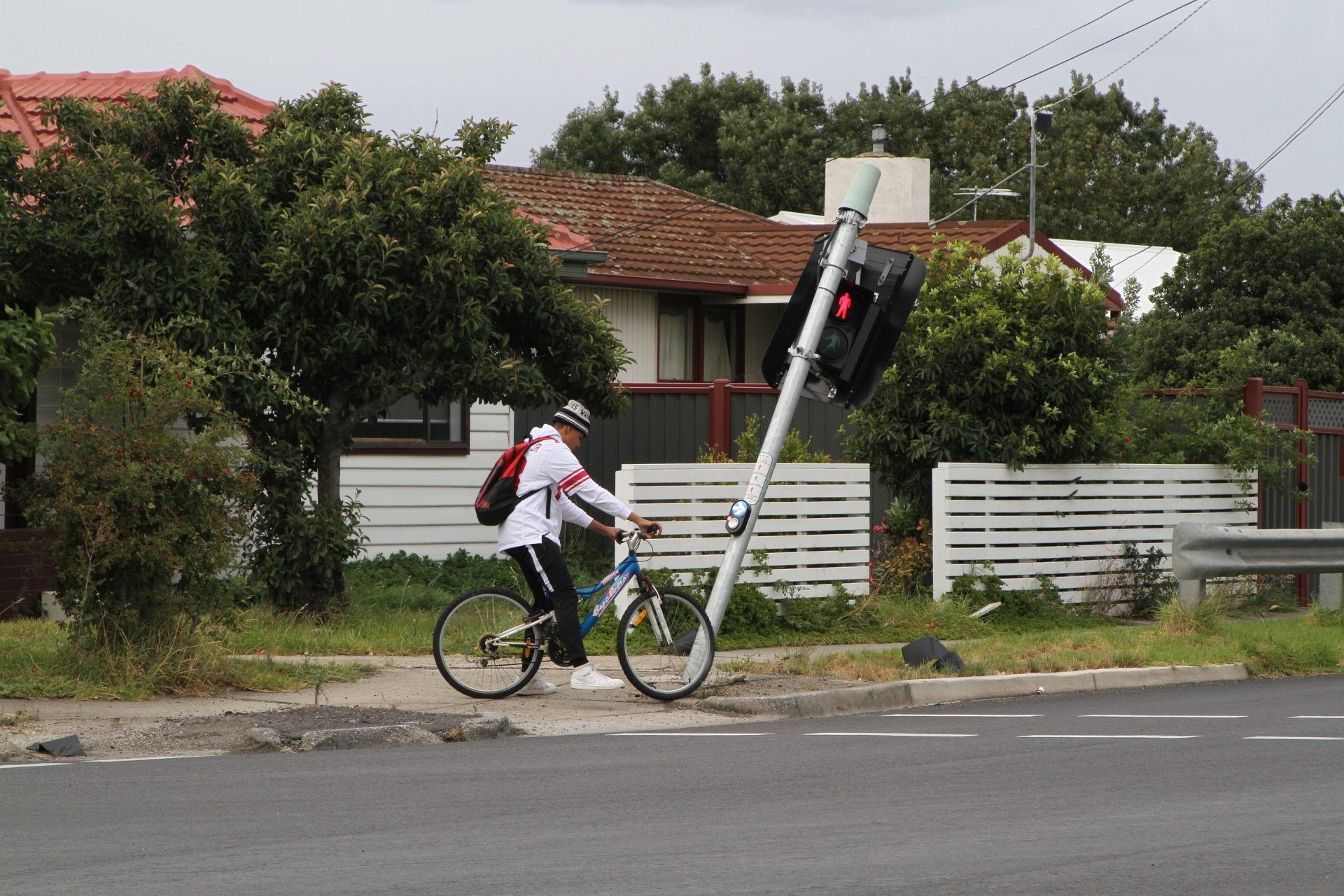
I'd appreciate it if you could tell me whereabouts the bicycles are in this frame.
[431,525,716,703]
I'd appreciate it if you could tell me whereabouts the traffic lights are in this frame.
[834,276,875,331]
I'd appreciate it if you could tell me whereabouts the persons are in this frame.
[498,399,662,695]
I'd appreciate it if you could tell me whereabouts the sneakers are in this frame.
[514,676,557,695]
[569,663,625,690]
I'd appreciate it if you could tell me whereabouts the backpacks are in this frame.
[474,433,560,526]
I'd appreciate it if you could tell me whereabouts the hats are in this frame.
[554,400,591,438]
